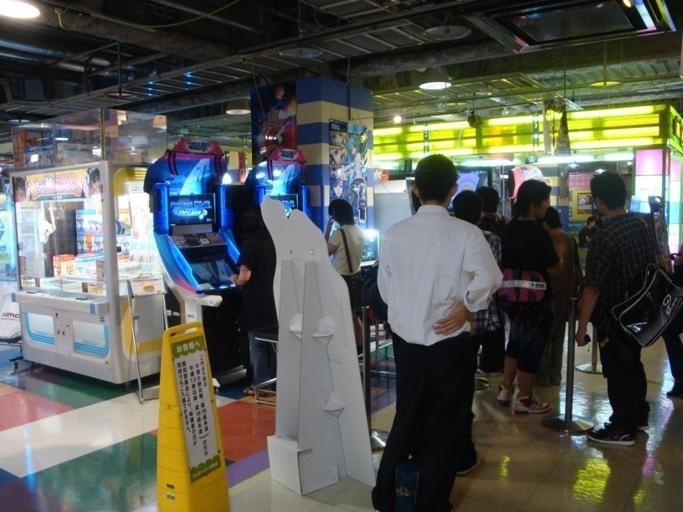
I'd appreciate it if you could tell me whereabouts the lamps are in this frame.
[418,67,452,91]
[278,29,323,59]
[425,16,472,41]
[225,99,251,115]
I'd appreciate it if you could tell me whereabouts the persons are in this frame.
[206,182,275,389]
[42,218,76,276]
[573,173,657,445]
[404,178,605,478]
[324,198,364,355]
[370,155,505,509]
[660,244,682,399]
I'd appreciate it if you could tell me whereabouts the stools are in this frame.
[252,334,279,407]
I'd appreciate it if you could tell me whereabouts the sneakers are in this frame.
[472,363,500,390]
[507,393,554,416]
[665,379,683,399]
[453,456,484,475]
[583,413,649,449]
[241,385,271,396]
[495,380,515,404]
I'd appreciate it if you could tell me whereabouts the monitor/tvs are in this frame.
[448,167,491,212]
[181,249,240,288]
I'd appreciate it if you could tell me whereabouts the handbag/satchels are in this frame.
[363,263,389,327]
[339,269,361,307]
[606,262,683,351]
[492,262,553,316]
